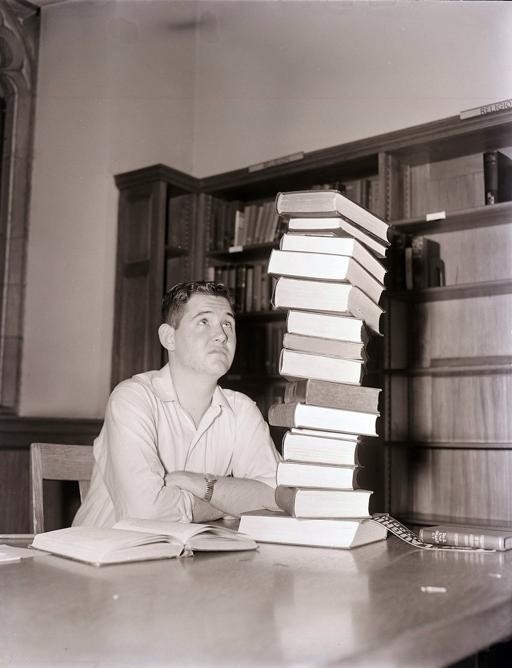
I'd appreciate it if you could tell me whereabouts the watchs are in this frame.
[200,472,217,503]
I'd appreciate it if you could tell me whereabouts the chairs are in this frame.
[31,443,93,534]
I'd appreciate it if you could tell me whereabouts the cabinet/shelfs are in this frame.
[108,99,512,529]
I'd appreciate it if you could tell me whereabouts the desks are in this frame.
[0,518,512,668]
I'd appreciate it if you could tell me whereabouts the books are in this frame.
[419,522,512,551]
[275,486,373,519]
[484,149,512,208]
[282,428,361,468]
[267,190,390,438]
[29,516,260,566]
[237,508,387,548]
[412,235,445,289]
[405,247,415,288]
[206,180,289,312]
[275,461,361,491]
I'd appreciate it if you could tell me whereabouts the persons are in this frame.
[70,279,284,529]
